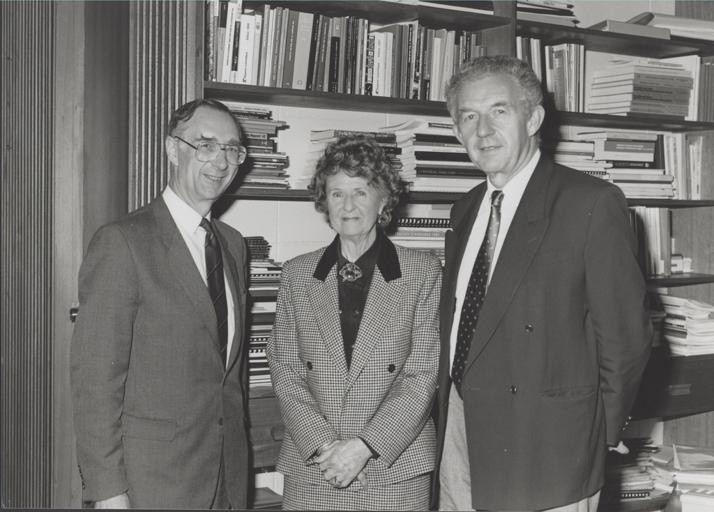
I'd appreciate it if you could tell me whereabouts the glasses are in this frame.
[172,133,246,167]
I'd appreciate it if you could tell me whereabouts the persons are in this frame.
[67,99,253,512]
[437,54,656,512]
[264,133,444,512]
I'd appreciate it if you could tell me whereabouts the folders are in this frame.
[586,20,671,41]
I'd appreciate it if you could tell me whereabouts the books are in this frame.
[224,101,487,194]
[620,418,713,512]
[385,201,461,269]
[629,206,714,358]
[552,125,705,201]
[240,234,285,388]
[205,1,714,123]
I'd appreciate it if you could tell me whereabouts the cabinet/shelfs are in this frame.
[127,0,714,512]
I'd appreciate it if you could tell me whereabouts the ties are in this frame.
[199,218,228,369]
[454,190,504,394]
